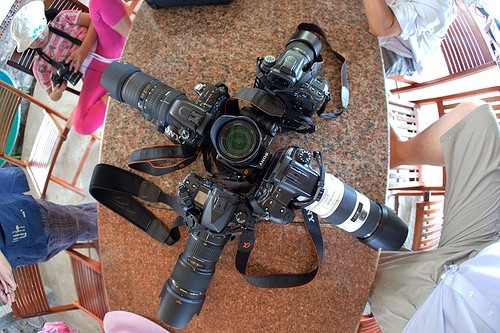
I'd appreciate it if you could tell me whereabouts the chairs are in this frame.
[11,242,108,324]
[388,86,500,191]
[388,191,445,252]
[386,0,497,95]
[7,0,90,96]
[0,78,103,201]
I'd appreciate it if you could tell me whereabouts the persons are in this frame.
[363,0,457,76]
[10,0,133,134]
[0,166,99,304]
[368,97,500,333]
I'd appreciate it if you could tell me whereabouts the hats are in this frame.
[10,0,47,52]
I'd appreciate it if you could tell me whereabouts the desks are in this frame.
[97,0,391,333]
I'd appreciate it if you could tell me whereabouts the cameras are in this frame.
[51,61,82,86]
[100,29,408,330]
